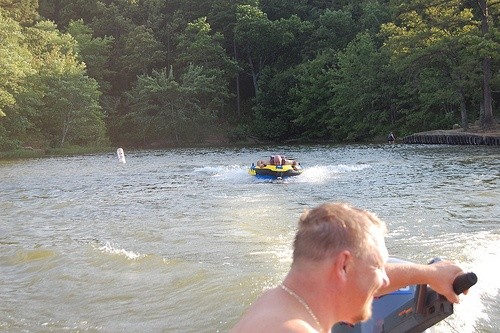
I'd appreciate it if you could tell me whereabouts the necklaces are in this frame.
[278,283,323,333]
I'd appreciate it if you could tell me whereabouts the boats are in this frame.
[250,156,303,181]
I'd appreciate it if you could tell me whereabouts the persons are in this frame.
[388,131,396,145]
[257,155,297,168]
[227,201,470,333]
